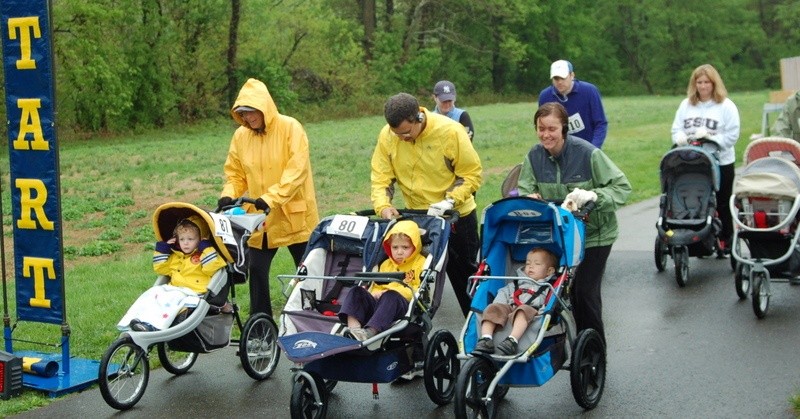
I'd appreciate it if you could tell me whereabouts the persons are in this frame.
[337,221,427,351]
[539,60,609,150]
[216,77,321,357]
[672,63,800,259]
[432,82,476,200]
[370,92,483,384]
[518,102,632,393]
[129,216,226,333]
[475,247,559,355]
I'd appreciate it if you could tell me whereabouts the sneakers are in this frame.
[343,328,369,341]
[497,336,518,356]
[474,337,495,354]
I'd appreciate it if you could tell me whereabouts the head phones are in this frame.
[535,103,570,134]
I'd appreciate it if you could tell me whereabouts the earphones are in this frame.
[418,110,425,123]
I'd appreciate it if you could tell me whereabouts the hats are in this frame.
[550,60,573,79]
[234,106,255,113]
[433,80,456,102]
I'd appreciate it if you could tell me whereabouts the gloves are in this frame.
[218,196,234,210]
[427,199,455,216]
[561,198,577,213]
[675,131,688,145]
[566,187,598,209]
[255,197,269,211]
[696,129,711,139]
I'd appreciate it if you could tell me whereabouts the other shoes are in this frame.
[236,339,273,358]
[129,319,160,332]
[716,248,730,259]
[400,361,425,381]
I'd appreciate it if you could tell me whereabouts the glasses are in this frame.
[388,116,418,137]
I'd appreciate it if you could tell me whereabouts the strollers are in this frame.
[729,137,800,319]
[654,137,732,288]
[277,206,460,419]
[98,197,281,411]
[453,196,606,419]
